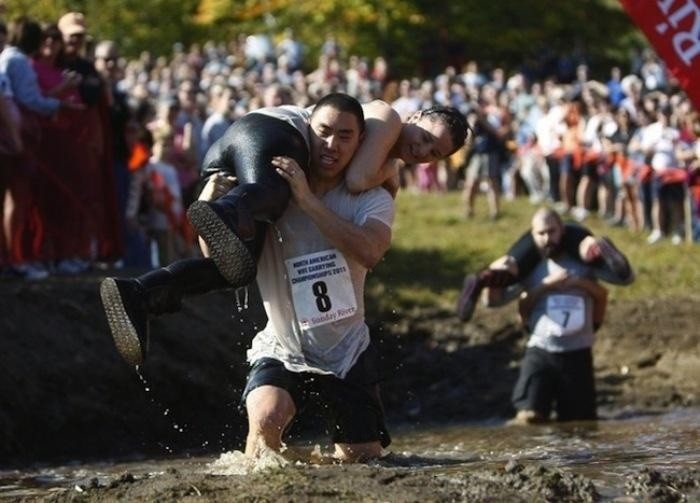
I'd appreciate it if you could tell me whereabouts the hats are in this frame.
[57,11,87,38]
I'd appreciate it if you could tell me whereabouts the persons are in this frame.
[455,216,635,335]
[98,96,470,368]
[194,91,391,465]
[479,204,602,426]
[393,48,699,244]
[2,0,440,280]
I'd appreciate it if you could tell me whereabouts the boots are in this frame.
[186,196,256,289]
[596,237,630,280]
[455,270,500,322]
[99,276,184,364]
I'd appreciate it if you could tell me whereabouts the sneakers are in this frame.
[7,253,125,280]
